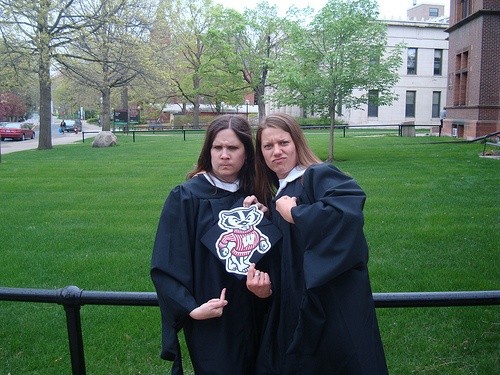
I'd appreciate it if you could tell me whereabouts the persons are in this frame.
[60,119,66,134]
[440,107,447,126]
[247,113,388,375]
[149,114,273,375]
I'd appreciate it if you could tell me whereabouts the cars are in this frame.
[0,118,35,141]
[64,119,82,132]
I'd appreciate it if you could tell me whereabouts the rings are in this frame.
[255,273,261,276]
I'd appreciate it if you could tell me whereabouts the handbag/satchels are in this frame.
[59,127,62,133]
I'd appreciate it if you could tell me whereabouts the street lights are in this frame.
[245,99,249,121]
[221,102,225,115]
